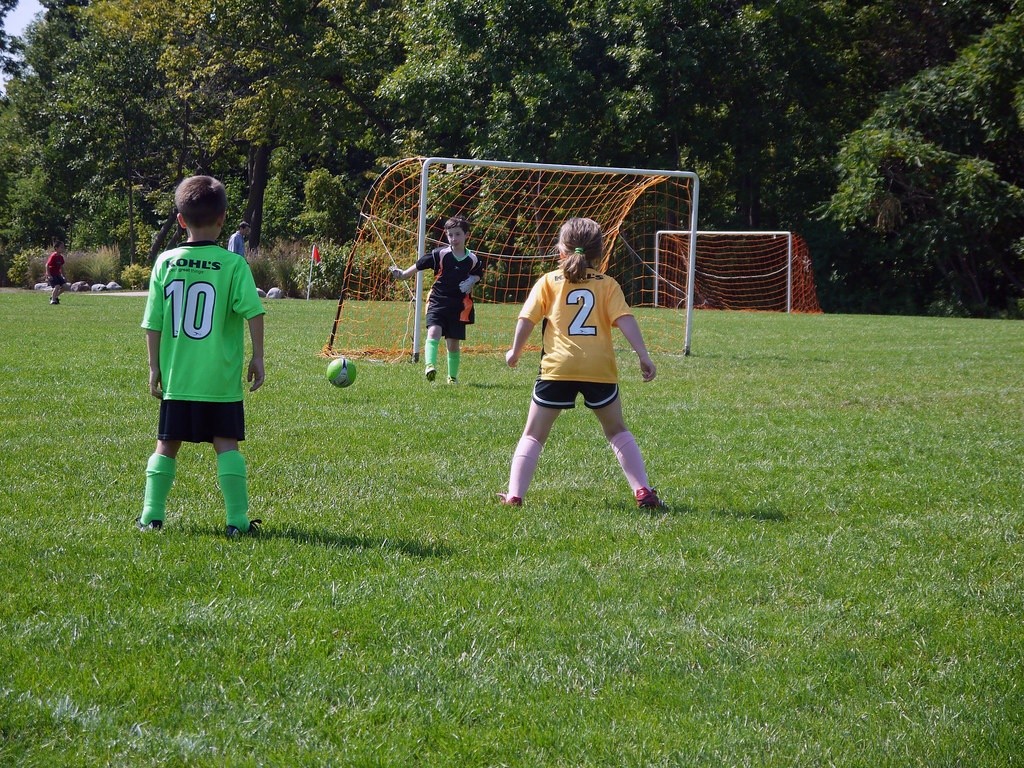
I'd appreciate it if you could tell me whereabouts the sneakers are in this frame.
[497,492,522,507]
[226,523,258,539]
[425,367,437,384]
[635,487,669,511]
[447,377,458,385]
[132,517,163,531]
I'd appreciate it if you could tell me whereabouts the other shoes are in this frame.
[50,300,57,304]
[50,297,59,303]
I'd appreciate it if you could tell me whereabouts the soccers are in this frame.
[326,358,357,388]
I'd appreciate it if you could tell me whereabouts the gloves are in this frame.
[458,277,477,294]
[391,269,407,280]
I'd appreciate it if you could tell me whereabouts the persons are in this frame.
[131,175,263,538]
[45,241,66,303]
[389,217,483,383]
[495,217,659,510]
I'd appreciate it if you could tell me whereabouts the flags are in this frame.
[313,247,321,265]
[227,221,250,257]
